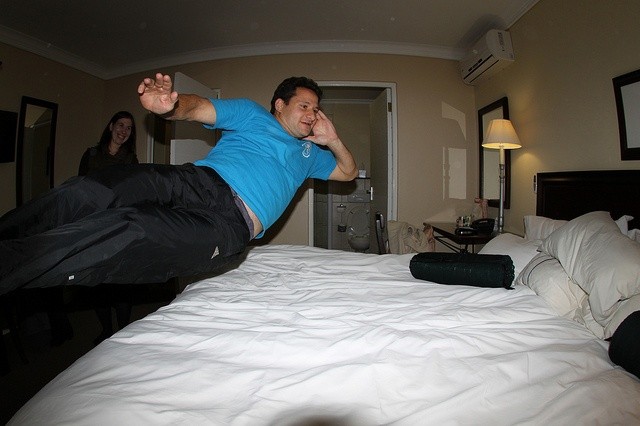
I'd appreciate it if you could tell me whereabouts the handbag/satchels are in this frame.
[398,222,435,254]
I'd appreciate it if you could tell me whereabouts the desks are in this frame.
[422,219,525,261]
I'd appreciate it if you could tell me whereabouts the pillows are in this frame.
[537,210,640,340]
[475,231,540,286]
[524,213,639,247]
[512,251,609,341]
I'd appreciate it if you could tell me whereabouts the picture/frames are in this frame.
[609,68,640,161]
[476,94,512,210]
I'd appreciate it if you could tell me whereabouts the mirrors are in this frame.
[14,93,58,206]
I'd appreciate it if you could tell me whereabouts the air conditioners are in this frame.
[457,29,517,86]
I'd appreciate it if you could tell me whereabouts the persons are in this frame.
[80,111,139,172]
[1,72,358,348]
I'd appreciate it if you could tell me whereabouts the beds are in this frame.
[5,170,640,425]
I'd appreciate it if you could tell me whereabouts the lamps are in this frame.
[481,119,522,236]
